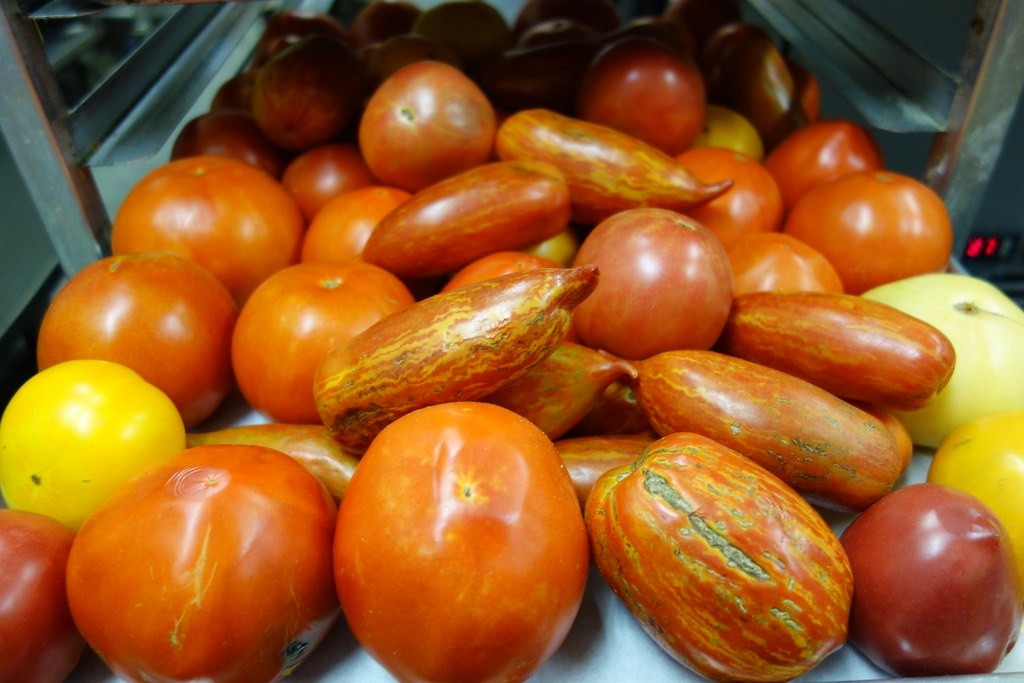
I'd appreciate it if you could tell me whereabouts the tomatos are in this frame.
[0,0,1018,682]
